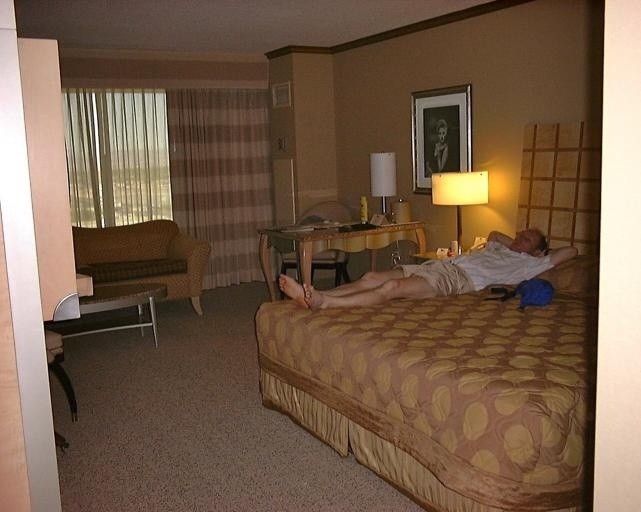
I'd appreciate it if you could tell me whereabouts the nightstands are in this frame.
[408,250,470,263]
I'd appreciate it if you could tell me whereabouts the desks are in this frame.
[48,281,167,347]
[255,216,426,305]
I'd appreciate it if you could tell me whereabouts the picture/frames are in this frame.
[409,82,472,196]
[270,81,294,107]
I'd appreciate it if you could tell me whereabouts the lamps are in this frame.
[368,151,395,213]
[430,171,488,248]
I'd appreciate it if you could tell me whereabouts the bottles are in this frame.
[360,196,368,224]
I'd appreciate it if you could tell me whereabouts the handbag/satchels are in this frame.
[514,278,552,308]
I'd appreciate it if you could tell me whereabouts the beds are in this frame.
[249,118,601,512]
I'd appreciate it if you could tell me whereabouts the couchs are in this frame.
[71,220,209,316]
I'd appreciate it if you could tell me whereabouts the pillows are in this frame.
[536,251,596,302]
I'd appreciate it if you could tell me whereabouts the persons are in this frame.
[277,229,579,311]
[424,118,457,177]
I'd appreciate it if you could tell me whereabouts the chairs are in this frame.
[279,198,351,300]
